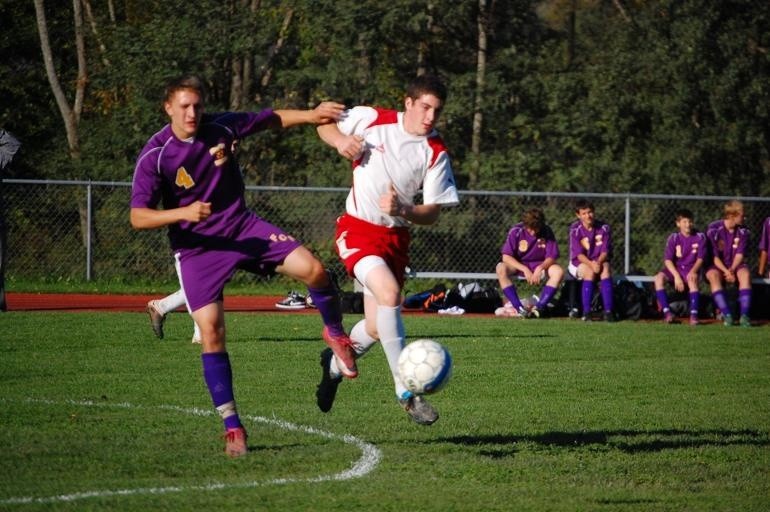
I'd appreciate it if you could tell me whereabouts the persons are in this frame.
[146,285,204,347]
[129,75,360,460]
[756,216,770,279]
[494,205,565,319]
[704,199,754,328]
[314,75,462,428]
[568,198,619,325]
[653,208,709,327]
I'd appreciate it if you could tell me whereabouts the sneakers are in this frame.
[316,347,343,413]
[396,390,439,425]
[146,299,167,340]
[603,310,618,322]
[438,305,466,315]
[663,310,675,324]
[274,289,306,310]
[739,314,754,329]
[321,325,358,378]
[518,305,545,319]
[689,313,699,326]
[224,426,249,459]
[581,311,592,321]
[722,313,733,327]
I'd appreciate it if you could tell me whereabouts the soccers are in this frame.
[397,339,452,396]
